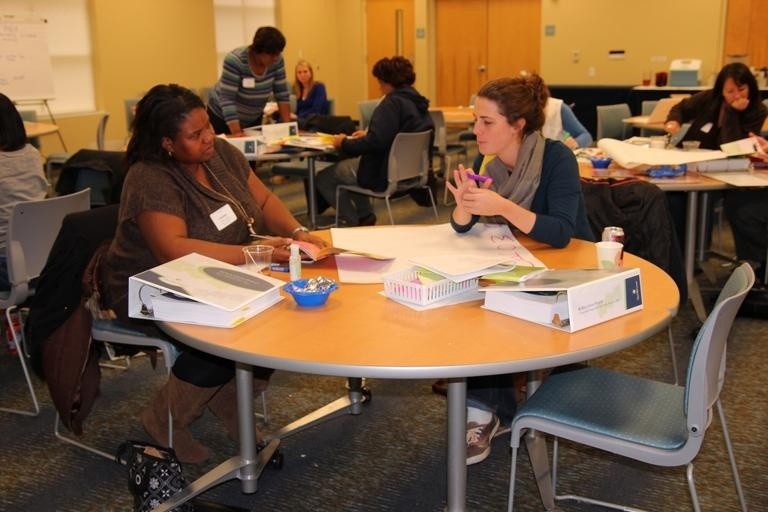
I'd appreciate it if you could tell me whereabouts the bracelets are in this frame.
[291,226,309,239]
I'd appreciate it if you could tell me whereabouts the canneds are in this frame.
[602,226,625,268]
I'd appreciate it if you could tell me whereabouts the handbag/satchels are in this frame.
[410,170,437,207]
[123,440,192,512]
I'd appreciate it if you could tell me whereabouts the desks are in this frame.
[155,225,680,512]
[632,82,710,113]
[235,122,369,229]
[22,117,58,140]
[572,140,768,325]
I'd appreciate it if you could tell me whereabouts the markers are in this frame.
[466,173,495,185]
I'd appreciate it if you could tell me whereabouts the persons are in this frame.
[446,73,596,466]
[315,55,439,226]
[0,93,52,293]
[663,61,768,151]
[205,26,291,137]
[722,132,768,292]
[538,97,594,152]
[289,61,329,131]
[101,84,331,465]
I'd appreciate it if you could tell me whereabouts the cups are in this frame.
[656,72,667,87]
[642,71,651,85]
[241,245,274,277]
[595,242,624,272]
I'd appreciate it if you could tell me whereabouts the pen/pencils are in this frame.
[250,234,271,240]
[271,266,289,273]
[561,130,571,143]
[667,124,675,130]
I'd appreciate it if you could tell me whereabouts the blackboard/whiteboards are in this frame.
[0,14,102,119]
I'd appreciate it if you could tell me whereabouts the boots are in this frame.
[142,367,223,464]
[206,376,268,443]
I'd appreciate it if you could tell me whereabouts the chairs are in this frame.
[508,262,756,512]
[641,99,659,137]
[429,110,468,205]
[199,87,215,106]
[619,116,669,140]
[333,127,440,226]
[124,99,139,136]
[1,186,92,418]
[20,110,41,150]
[327,98,336,116]
[762,96,768,109]
[359,100,382,131]
[44,113,110,185]
[596,103,633,143]
[54,211,268,469]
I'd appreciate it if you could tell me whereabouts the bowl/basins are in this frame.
[682,140,701,150]
[284,279,337,306]
[719,138,754,157]
[590,157,612,169]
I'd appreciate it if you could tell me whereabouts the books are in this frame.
[686,157,752,172]
[254,234,398,264]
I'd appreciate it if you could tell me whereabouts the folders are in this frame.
[477,267,645,334]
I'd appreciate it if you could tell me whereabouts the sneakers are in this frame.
[361,214,376,226]
[466,412,511,465]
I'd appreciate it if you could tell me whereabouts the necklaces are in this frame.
[193,160,257,235]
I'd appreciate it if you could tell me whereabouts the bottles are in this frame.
[289,244,302,282]
[5,317,23,357]
